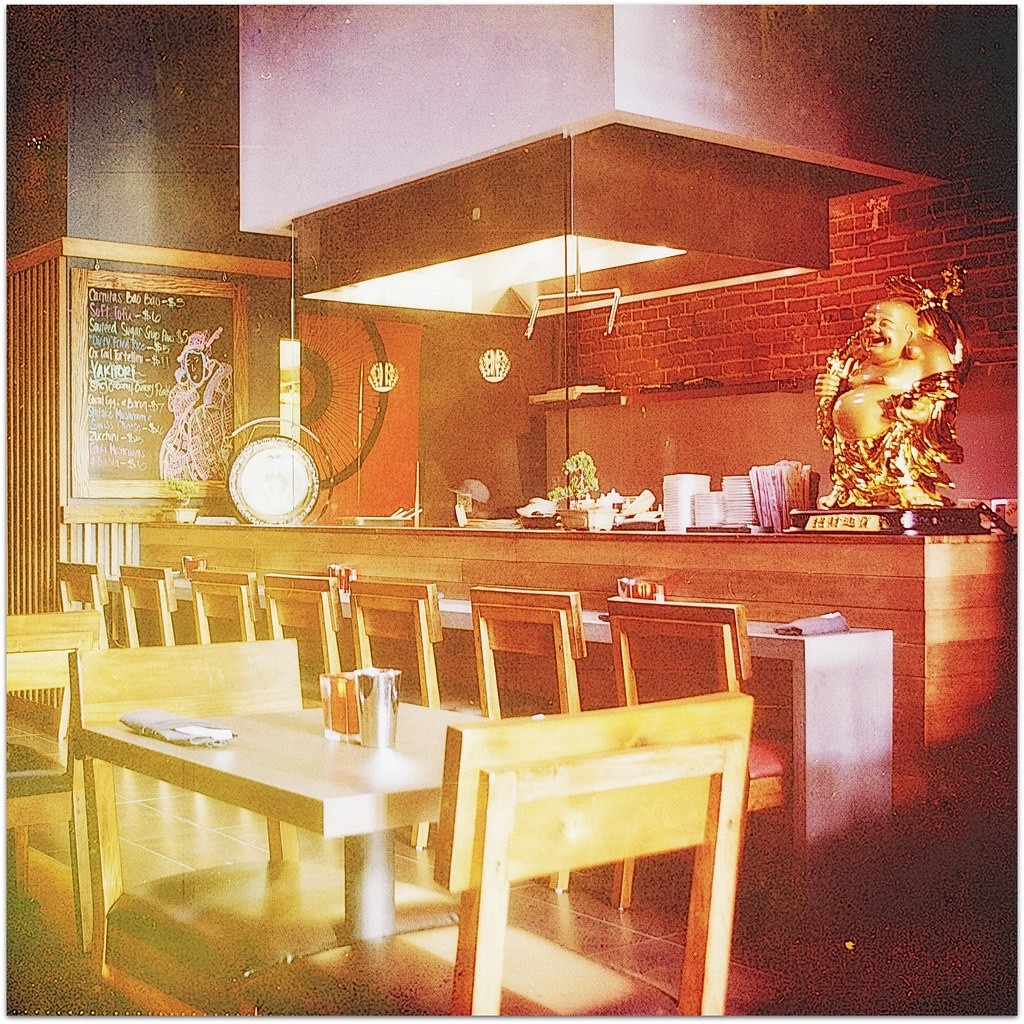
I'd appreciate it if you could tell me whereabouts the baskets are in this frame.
[556,510,591,530]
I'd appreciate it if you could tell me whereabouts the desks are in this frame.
[107,574,895,878]
[81,701,487,943]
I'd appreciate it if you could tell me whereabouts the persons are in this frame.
[815,300,962,506]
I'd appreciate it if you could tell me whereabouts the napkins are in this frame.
[118,708,240,747]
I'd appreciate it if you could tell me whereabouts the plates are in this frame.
[662,473,758,533]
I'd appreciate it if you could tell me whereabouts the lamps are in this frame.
[367,361,400,393]
[477,348,512,383]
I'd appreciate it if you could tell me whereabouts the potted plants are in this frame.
[166,477,201,524]
[546,450,614,532]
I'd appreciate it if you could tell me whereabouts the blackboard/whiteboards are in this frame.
[71,268,248,500]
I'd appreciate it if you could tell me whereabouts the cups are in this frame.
[355,668,402,749]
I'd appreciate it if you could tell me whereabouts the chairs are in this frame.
[7,560,791,1016]
